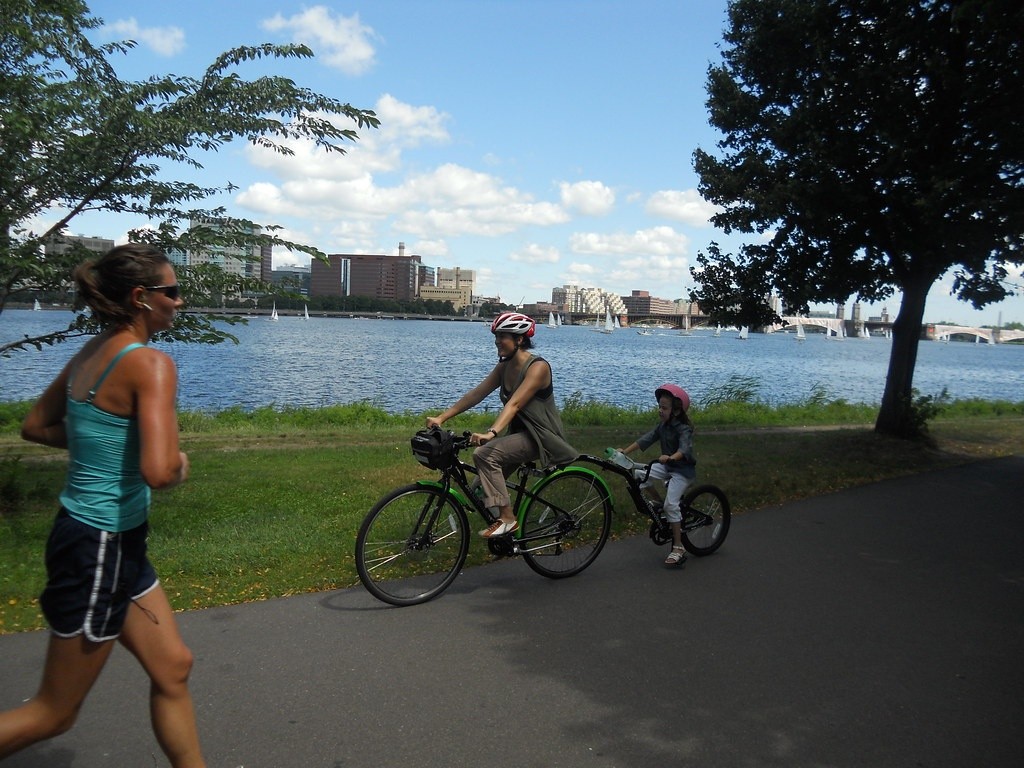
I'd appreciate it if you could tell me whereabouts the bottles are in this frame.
[475,485,499,517]
[605,447,633,469]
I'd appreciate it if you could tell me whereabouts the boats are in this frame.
[678,331,692,336]
[241,315,258,319]
[482,324,492,328]
[638,329,654,336]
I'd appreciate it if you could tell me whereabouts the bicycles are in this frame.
[353,427,732,605]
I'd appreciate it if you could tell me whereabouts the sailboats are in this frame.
[735,325,750,340]
[712,323,722,337]
[794,319,806,340]
[986,332,996,347]
[29,300,42,311]
[263,300,279,322]
[589,311,621,334]
[546,311,562,329]
[823,321,893,343]
[940,330,951,344]
[300,306,313,321]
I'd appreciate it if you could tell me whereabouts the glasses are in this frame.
[135,285,178,300]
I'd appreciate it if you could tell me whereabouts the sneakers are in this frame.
[492,554,502,561]
[478,517,518,538]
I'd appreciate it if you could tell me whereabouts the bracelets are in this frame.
[487,429,497,438]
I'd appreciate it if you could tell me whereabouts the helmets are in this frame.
[655,383,690,413]
[491,312,535,338]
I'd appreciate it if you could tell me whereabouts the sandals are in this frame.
[649,500,665,508]
[665,544,687,564]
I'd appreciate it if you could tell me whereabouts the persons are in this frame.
[425,312,579,561]
[616,383,697,563]
[0,243,206,768]
